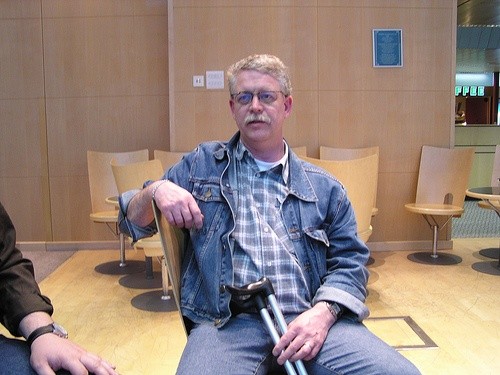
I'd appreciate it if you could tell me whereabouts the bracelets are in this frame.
[152,179,171,199]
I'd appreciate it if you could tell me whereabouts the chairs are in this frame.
[86,149,193,338]
[405,144,500,275]
[291,143,381,266]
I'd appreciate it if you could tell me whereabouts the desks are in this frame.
[465,186,500,276]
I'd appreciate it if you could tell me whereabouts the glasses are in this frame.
[233,90,285,105]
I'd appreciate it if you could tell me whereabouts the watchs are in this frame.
[325,300,342,321]
[27,322,68,348]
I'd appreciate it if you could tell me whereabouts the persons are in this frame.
[117,52,421,375]
[0,202,117,375]
